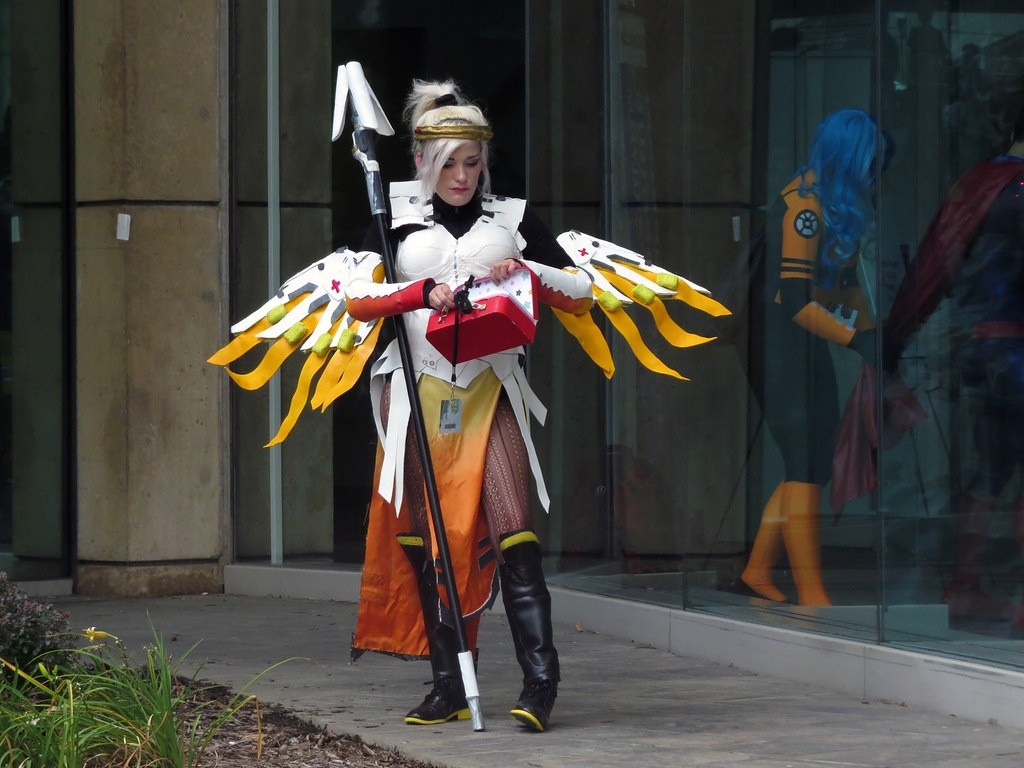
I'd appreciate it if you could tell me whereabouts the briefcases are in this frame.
[425,267,538,366]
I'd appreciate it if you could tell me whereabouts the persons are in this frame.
[934,100,1024,639]
[344,79,593,732]
[732,107,902,607]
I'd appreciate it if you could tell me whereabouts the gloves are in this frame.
[850,327,901,372]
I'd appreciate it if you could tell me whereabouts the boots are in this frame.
[780,479,830,608]
[492,528,562,733]
[397,531,481,724]
[740,478,785,603]
